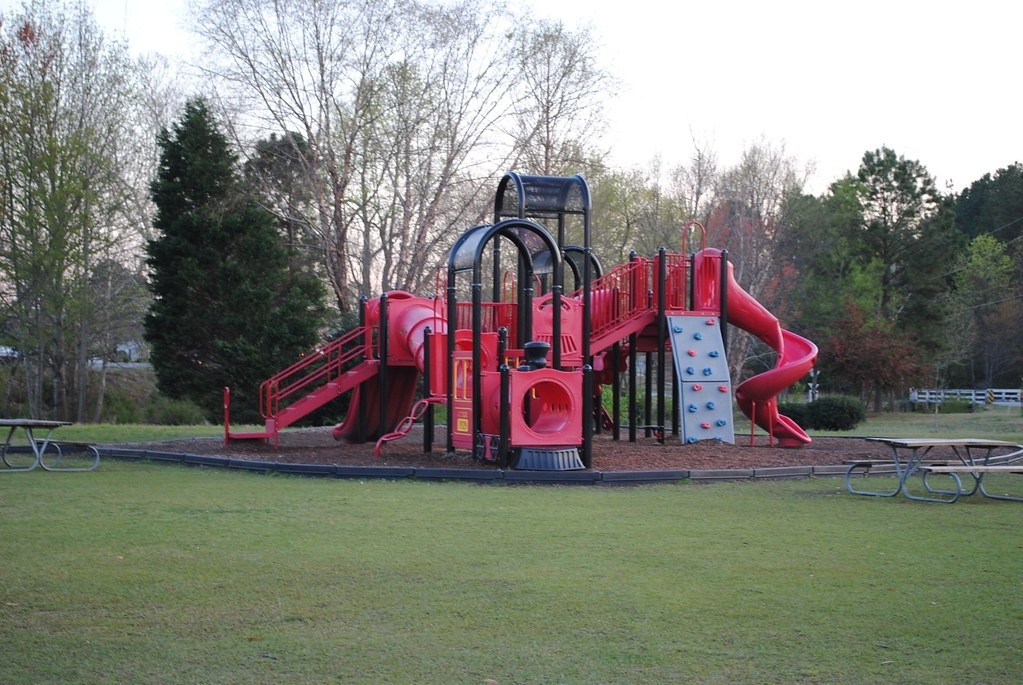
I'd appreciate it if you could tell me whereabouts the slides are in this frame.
[331,247,819,471]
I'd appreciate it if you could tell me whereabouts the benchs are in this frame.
[0,418,99,472]
[842,438,1023,504]
